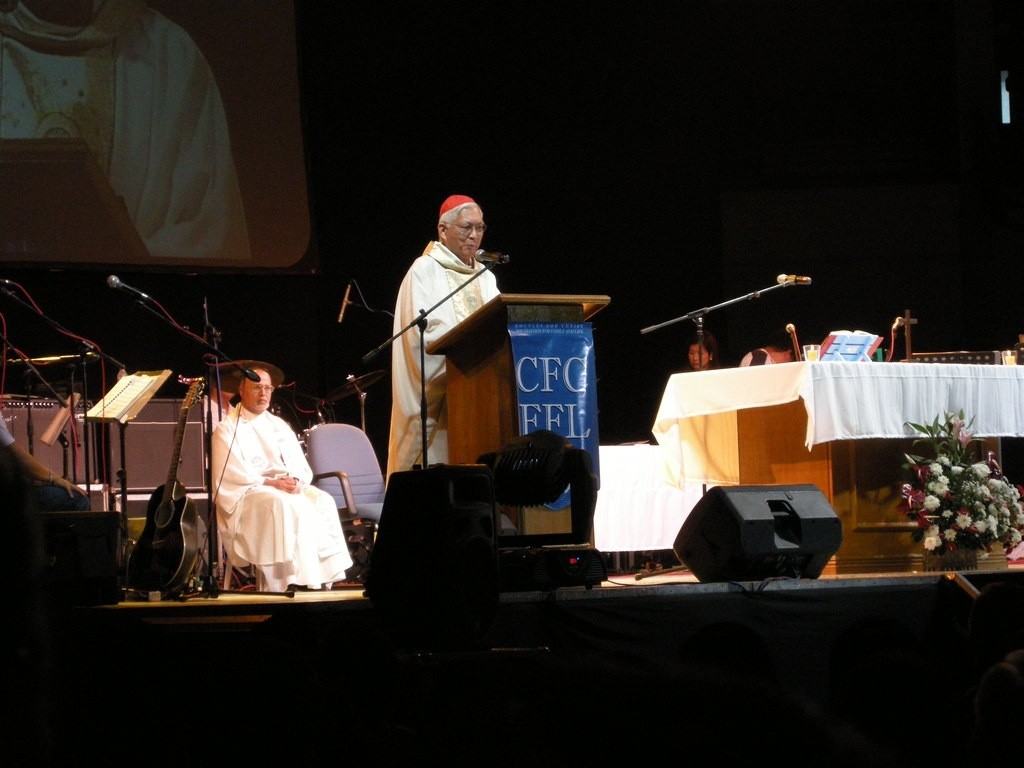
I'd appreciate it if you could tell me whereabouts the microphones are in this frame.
[776,274,812,286]
[474,249,510,264]
[0,279,13,284]
[337,284,351,322]
[107,275,152,302]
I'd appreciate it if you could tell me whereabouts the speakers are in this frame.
[0,397,208,610]
[364,462,499,650]
[673,484,843,585]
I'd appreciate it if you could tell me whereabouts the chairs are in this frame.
[307,424,386,581]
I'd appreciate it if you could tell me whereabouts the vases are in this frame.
[920,534,977,572]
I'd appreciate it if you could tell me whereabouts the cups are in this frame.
[1001,350,1018,365]
[803,344,820,362]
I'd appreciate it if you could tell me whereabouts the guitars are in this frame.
[128,382,205,592]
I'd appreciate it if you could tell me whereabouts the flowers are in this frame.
[902,412,1024,553]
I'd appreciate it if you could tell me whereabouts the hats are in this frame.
[439,194,475,218]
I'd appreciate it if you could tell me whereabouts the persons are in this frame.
[738,337,798,370]
[0,1,251,261]
[687,339,725,369]
[385,193,505,487]
[212,367,355,595]
[0,395,93,498]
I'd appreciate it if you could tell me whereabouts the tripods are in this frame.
[134,299,295,601]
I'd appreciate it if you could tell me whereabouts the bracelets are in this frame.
[48,467,55,486]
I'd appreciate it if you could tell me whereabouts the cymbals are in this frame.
[205,359,286,394]
[324,370,386,402]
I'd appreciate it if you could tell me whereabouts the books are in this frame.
[87,375,160,419]
